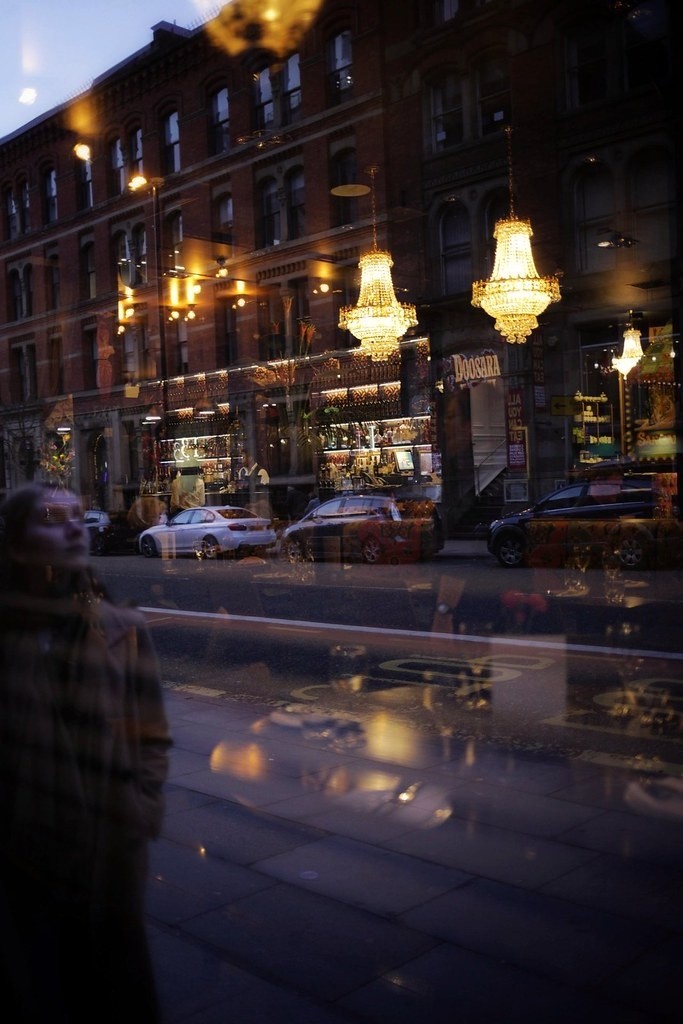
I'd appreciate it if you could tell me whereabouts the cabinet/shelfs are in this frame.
[573,402,614,466]
[140,399,442,513]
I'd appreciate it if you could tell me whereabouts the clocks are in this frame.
[471,130,562,345]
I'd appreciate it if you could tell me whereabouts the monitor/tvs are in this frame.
[395,451,415,474]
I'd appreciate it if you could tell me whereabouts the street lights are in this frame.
[128,175,171,520]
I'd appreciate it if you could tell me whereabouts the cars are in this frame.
[277,493,445,565]
[138,504,277,561]
[84,510,117,556]
[487,479,683,571]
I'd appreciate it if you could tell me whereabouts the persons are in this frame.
[0,487,171,1024]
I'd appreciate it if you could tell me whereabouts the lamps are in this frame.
[612,330,645,381]
[339,165,419,361]
